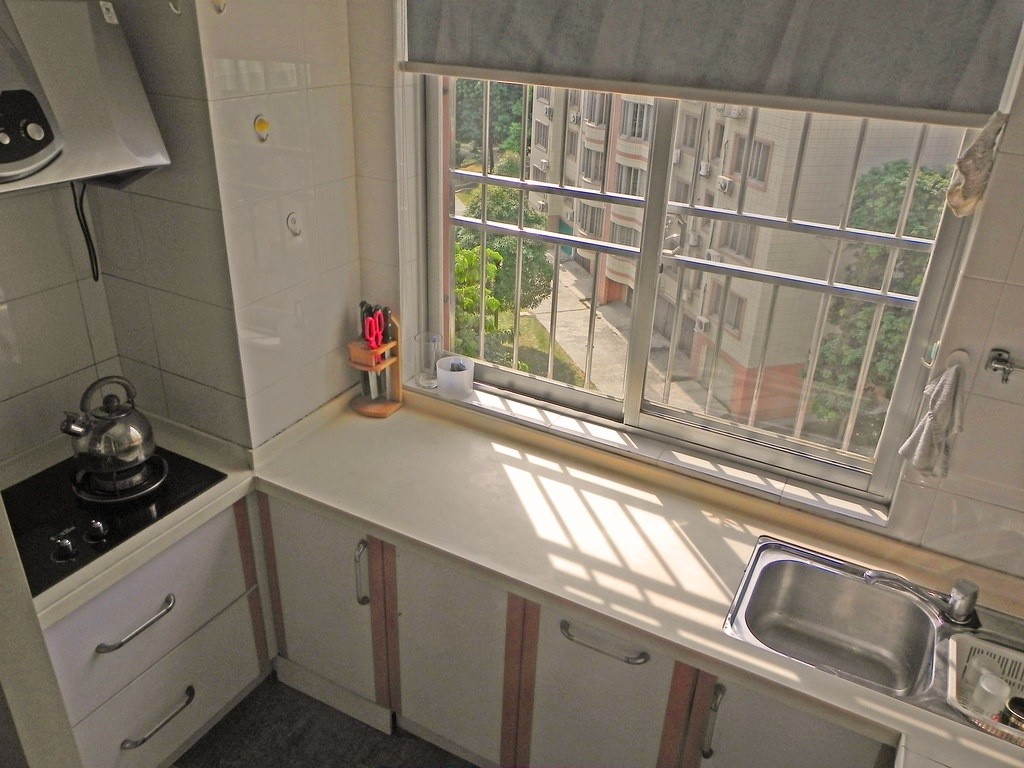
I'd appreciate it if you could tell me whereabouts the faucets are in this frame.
[859,566,981,625]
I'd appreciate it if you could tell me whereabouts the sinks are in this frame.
[723,534,947,708]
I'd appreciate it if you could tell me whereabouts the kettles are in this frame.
[60,375,157,473]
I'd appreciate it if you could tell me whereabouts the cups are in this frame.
[971,674,1011,722]
[414,332,442,389]
[435,354,478,401]
[962,653,1002,692]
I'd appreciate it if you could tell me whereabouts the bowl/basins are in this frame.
[1002,696,1024,733]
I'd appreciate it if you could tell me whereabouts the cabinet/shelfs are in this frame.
[257,490,527,768]
[519,593,699,765]
[679,663,897,768]
[38,485,283,768]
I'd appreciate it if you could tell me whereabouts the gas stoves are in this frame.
[0,442,232,600]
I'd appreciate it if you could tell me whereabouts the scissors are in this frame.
[365,311,384,396]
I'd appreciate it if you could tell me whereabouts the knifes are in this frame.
[356,300,394,402]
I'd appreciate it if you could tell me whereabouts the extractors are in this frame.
[0,0,172,281]
